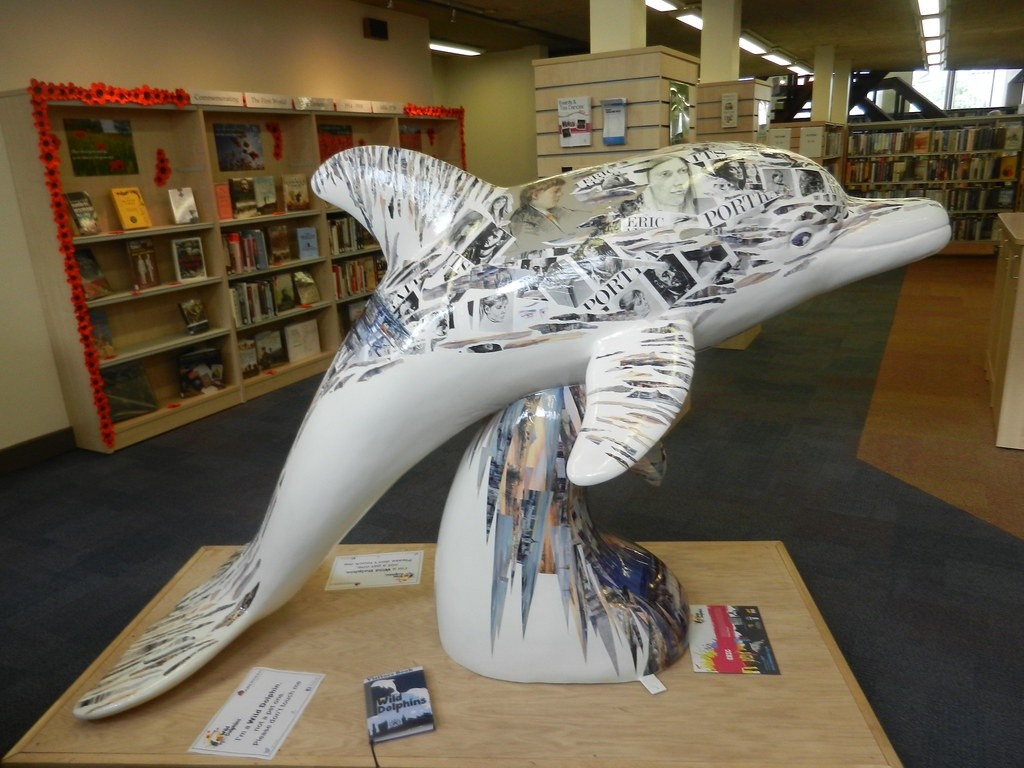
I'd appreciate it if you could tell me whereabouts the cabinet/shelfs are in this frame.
[844,114,1024,242]
[0,80,469,455]
[984,212,1024,451]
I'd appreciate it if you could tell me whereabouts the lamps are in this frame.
[646,0,815,76]
[430,40,485,57]
[916,0,951,74]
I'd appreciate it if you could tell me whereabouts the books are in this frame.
[359,666,434,744]
[825,121,1022,242]
[60,172,387,421]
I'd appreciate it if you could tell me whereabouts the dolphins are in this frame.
[74,143,955,723]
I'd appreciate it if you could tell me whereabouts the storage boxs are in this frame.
[283,318,321,363]
